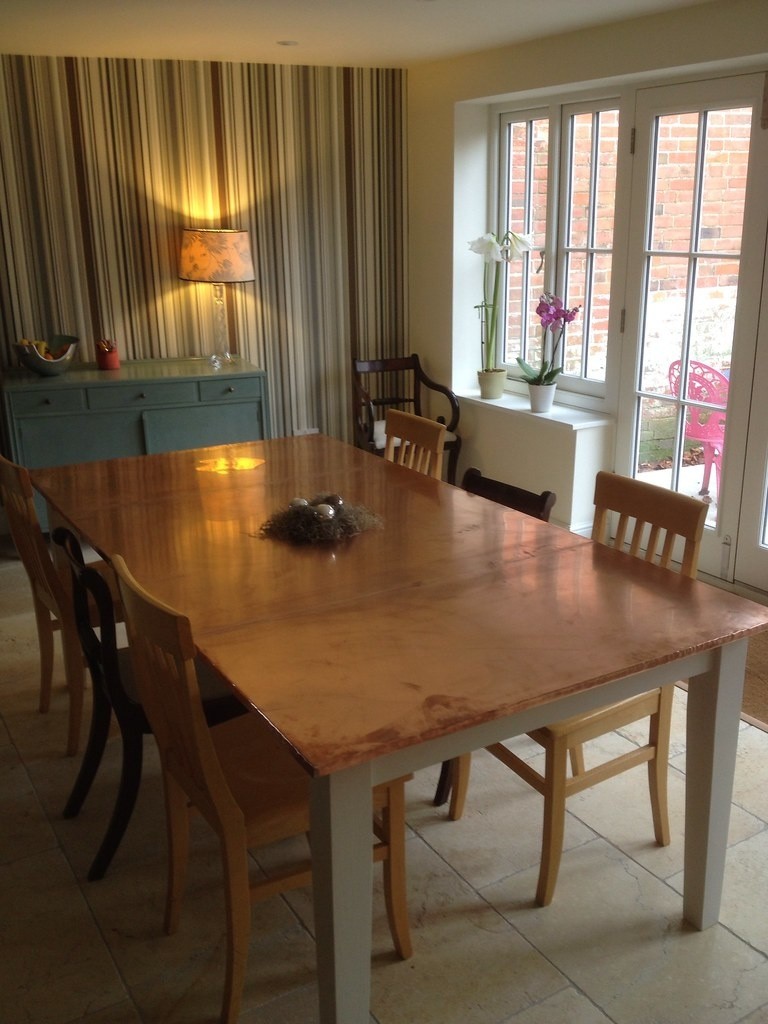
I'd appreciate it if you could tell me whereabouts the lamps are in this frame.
[178,229,256,368]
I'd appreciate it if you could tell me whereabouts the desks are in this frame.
[21,432,768,1022]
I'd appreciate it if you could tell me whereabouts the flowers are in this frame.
[513,291,582,387]
[468,229,534,372]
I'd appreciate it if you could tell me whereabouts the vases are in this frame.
[478,368,508,400]
[529,383,556,413]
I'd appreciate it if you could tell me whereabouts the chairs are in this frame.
[50,525,249,883]
[431,466,557,808]
[668,359,730,502]
[113,553,412,1023]
[445,470,709,911]
[350,353,462,487]
[0,454,126,757]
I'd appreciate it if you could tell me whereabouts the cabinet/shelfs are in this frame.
[1,352,272,537]
